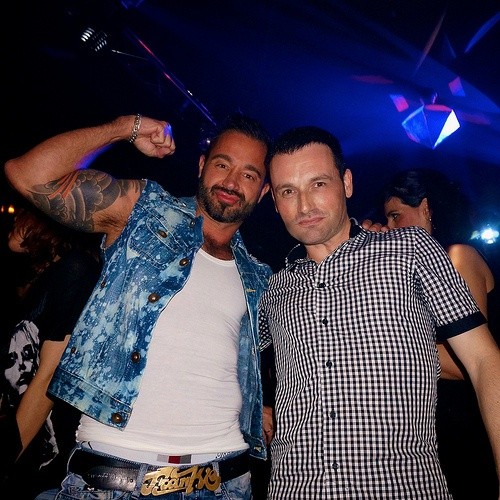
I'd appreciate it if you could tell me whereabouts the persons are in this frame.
[0,204,102,500]
[380,169,500,500]
[5,113,391,500]
[260,125,500,500]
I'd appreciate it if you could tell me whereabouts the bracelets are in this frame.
[128,113,141,142]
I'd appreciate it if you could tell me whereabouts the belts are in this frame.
[68,448,251,496]
